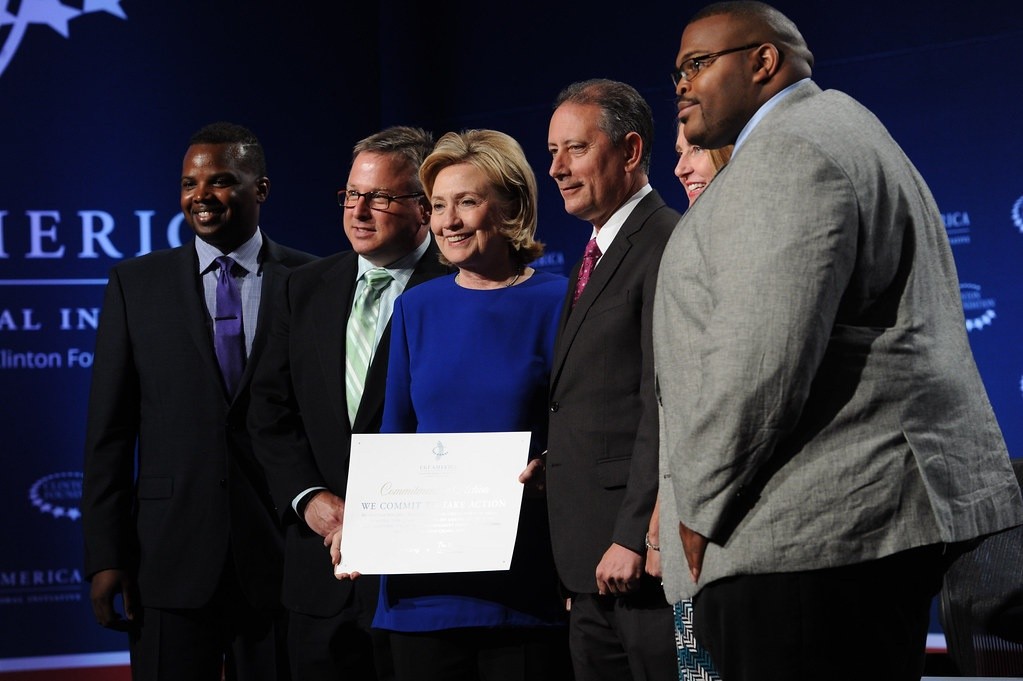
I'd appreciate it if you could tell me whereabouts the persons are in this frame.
[644,116,737,681]
[523,79,682,681]
[80,124,327,681]
[247,125,460,681]
[371,129,572,681]
[654,0,1023,681]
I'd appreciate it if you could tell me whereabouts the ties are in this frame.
[345,268,394,429]
[571,238,602,310]
[213,255,246,398]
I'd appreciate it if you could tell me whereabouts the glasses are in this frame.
[338,190,422,210]
[671,44,784,88]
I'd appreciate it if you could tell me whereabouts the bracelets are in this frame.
[645,533,659,551]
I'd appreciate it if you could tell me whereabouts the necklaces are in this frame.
[505,268,521,287]
